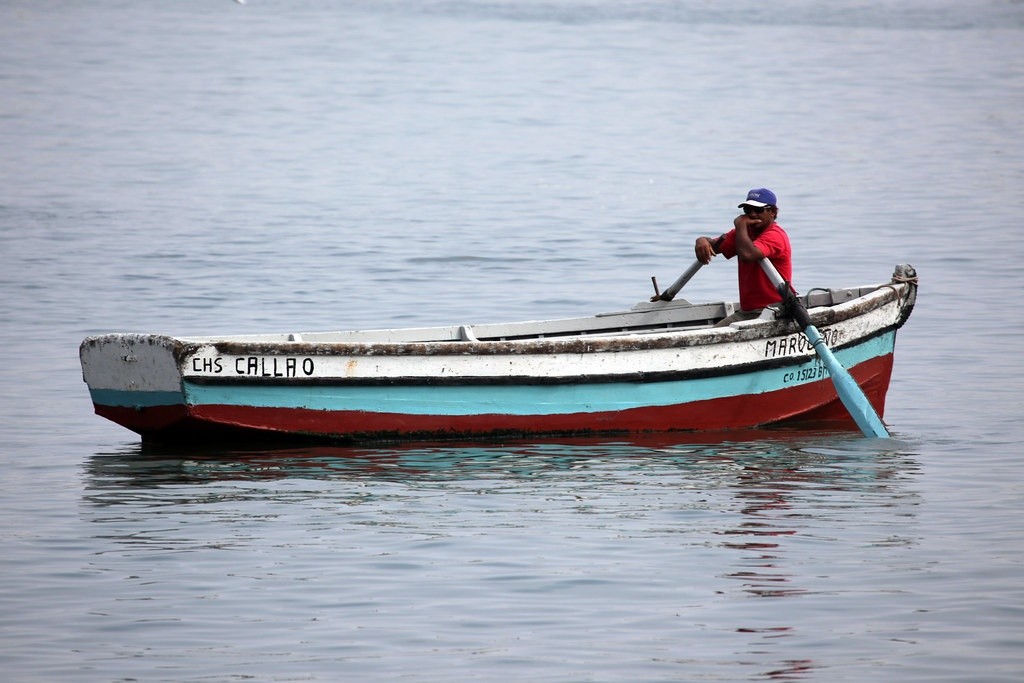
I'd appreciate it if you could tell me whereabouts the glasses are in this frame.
[743,205,771,214]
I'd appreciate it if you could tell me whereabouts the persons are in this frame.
[695,189,792,327]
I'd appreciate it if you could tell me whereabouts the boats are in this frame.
[79,263,918,457]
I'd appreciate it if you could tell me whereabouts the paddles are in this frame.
[661,233,727,301]
[759,256,890,438]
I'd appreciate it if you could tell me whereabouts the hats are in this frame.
[738,187,777,207]
